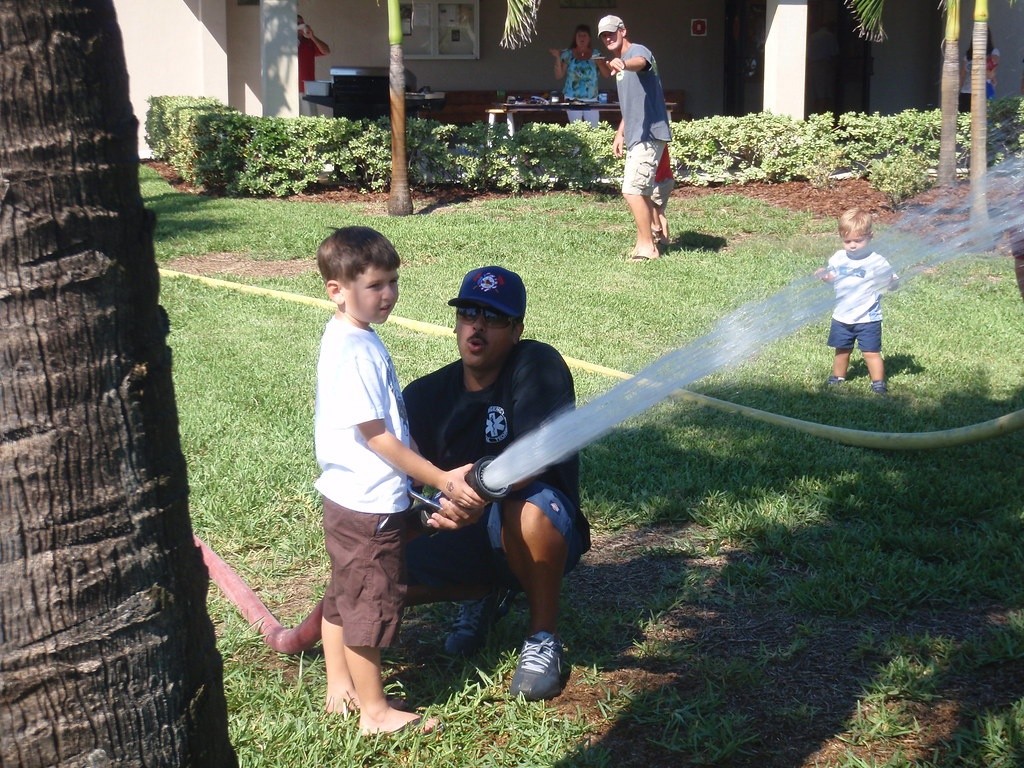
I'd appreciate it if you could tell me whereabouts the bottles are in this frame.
[549,88,561,106]
[496,86,505,106]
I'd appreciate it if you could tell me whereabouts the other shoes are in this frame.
[650,226,666,244]
[871,380,888,395]
[826,375,845,384]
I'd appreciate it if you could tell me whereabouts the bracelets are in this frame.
[621,59,626,69]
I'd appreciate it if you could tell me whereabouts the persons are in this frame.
[314,226,490,743]
[549,24,611,126]
[814,206,900,395]
[401,267,592,701]
[960,27,999,112]
[297,14,330,116]
[597,15,675,261]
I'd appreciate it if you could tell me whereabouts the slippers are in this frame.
[321,693,409,717]
[623,255,652,264]
[356,712,445,739]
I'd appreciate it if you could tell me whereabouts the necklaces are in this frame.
[578,50,586,56]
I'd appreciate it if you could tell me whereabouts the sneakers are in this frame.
[444,585,517,656]
[511,631,563,701]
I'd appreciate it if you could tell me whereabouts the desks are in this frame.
[484,102,678,148]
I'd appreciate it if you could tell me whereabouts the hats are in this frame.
[448,266,526,319]
[597,14,625,39]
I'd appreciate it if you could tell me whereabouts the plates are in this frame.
[590,57,606,60]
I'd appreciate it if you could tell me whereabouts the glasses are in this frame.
[456,301,516,328]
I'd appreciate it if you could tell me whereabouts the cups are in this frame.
[599,92,607,105]
